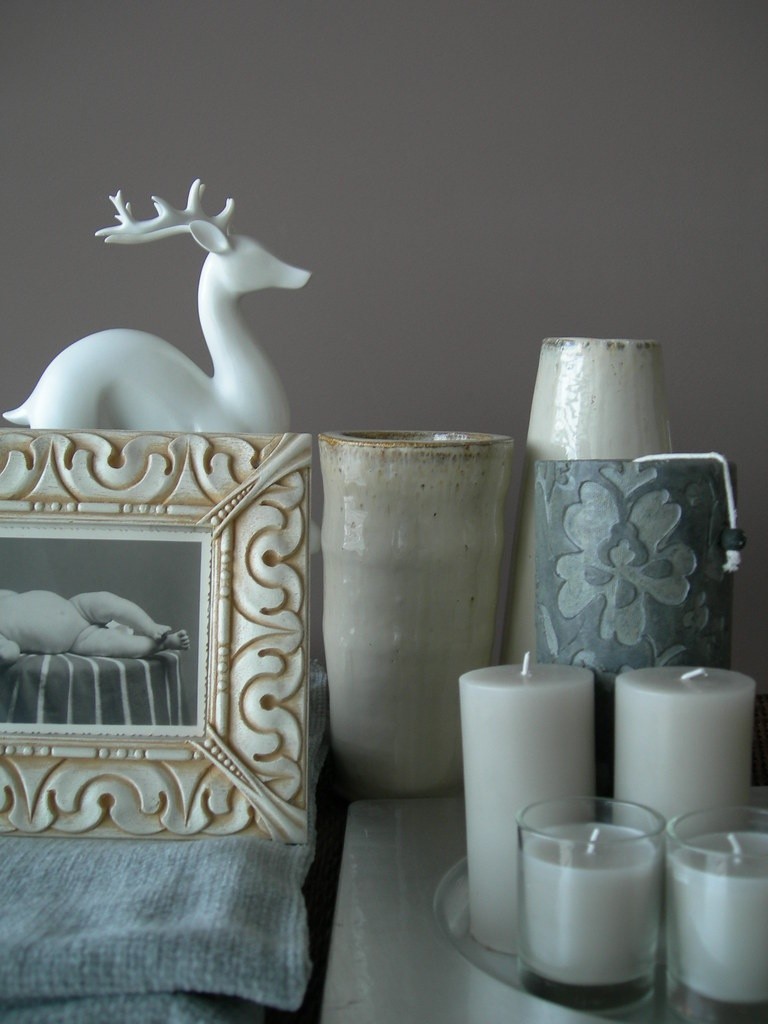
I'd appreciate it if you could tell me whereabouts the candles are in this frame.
[515,797,666,1017]
[656,805,768,1024]
[457,652,595,958]
[611,664,757,930]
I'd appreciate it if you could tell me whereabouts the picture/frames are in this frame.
[0,425,314,844]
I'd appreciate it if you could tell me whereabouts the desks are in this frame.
[318,795,673,1024]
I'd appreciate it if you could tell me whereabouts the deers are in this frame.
[2,178,316,436]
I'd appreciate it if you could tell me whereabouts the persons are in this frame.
[0,587,189,668]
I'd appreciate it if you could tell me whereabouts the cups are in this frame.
[665,806,768,1024]
[319,428,513,798]
[535,459,736,783]
[518,797,667,991]
